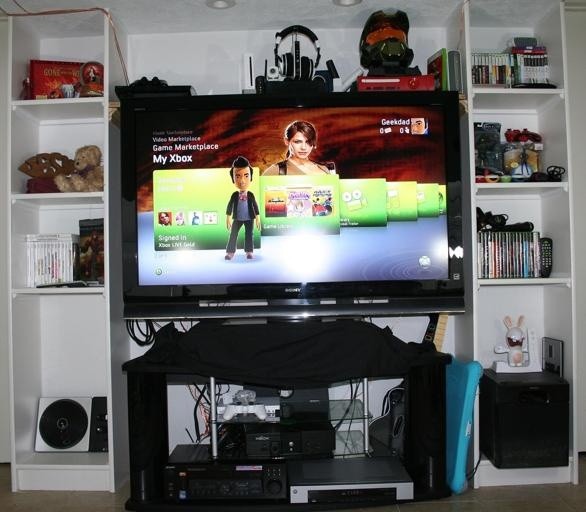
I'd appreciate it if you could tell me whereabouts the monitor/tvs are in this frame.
[120,91,464,318]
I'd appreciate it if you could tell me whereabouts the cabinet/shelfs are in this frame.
[122,353,452,512]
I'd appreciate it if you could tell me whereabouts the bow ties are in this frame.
[239,194,248,201]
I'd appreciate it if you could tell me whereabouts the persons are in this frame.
[260,120,334,177]
[224,156,262,261]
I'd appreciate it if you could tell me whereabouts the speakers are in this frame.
[478,368,570,470]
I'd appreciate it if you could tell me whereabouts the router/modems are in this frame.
[242,56,268,94]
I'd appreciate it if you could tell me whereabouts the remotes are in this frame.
[540,237,553,278]
[37,280,87,288]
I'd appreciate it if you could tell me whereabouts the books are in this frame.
[470,47,550,87]
[24,216,104,292]
[427,48,462,92]
[476,229,543,279]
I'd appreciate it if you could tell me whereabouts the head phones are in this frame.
[271,25,322,80]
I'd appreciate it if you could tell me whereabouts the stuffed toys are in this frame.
[55,145,104,193]
